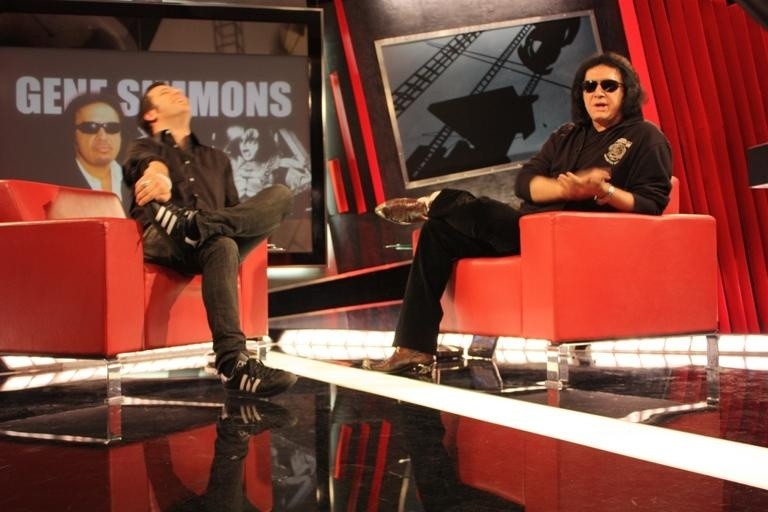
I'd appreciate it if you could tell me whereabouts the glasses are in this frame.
[581,79,625,93]
[73,121,121,135]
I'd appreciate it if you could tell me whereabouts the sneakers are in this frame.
[217,393,298,435]
[218,357,298,397]
[145,199,201,255]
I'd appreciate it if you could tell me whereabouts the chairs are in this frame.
[410,176,721,424]
[0,178,273,447]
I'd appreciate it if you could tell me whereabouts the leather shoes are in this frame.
[375,190,441,230]
[361,345,434,380]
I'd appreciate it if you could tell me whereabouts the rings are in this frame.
[141,181,151,187]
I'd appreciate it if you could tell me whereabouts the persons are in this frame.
[65,94,133,213]
[122,81,297,399]
[222,126,312,203]
[370,51,672,374]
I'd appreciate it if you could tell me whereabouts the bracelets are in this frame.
[594,183,614,206]
